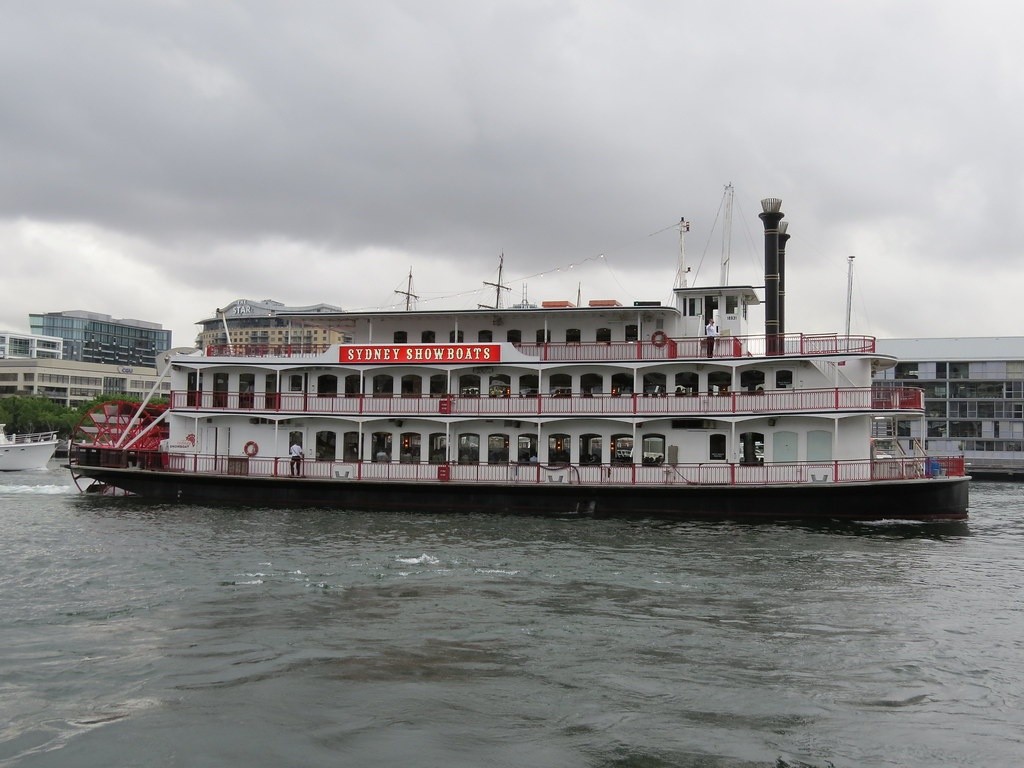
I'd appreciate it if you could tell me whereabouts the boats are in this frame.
[70,285,972,519]
[0,423,60,471]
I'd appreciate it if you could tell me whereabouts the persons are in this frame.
[376,449,388,463]
[464,389,477,398]
[584,390,592,397]
[554,390,561,397]
[675,387,682,396]
[289,442,302,476]
[401,451,411,463]
[529,452,538,461]
[462,452,470,464]
[519,392,523,398]
[590,455,597,464]
[432,451,444,462]
[706,319,720,358]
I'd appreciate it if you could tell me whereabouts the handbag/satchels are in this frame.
[299,454,303,459]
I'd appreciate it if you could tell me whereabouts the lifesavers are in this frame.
[651,330,669,348]
[244,441,259,457]
